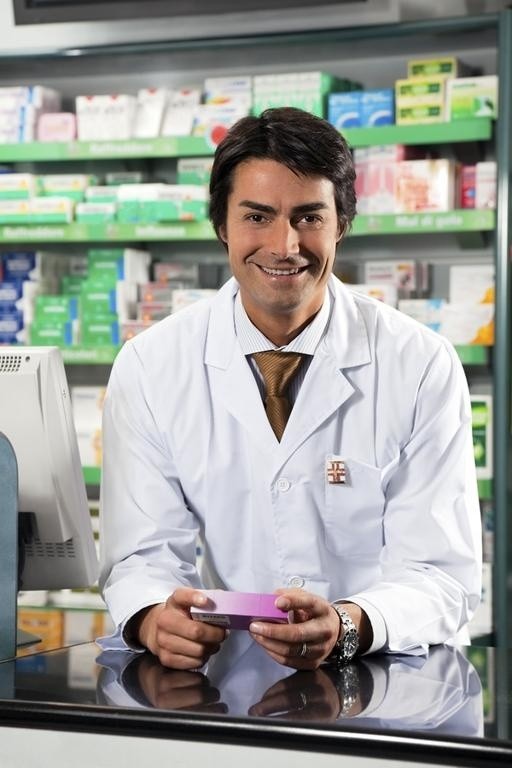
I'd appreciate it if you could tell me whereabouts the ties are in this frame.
[250,350,304,442]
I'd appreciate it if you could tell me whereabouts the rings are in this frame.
[297,691,307,711]
[300,643,307,656]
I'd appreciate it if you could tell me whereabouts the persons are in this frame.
[93,104,485,671]
[95,645,485,739]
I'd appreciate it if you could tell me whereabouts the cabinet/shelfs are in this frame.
[0,116,493,501]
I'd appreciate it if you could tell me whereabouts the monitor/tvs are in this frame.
[0,0,401,56]
[0,345,99,647]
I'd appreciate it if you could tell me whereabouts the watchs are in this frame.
[336,665,360,720]
[331,602,360,662]
